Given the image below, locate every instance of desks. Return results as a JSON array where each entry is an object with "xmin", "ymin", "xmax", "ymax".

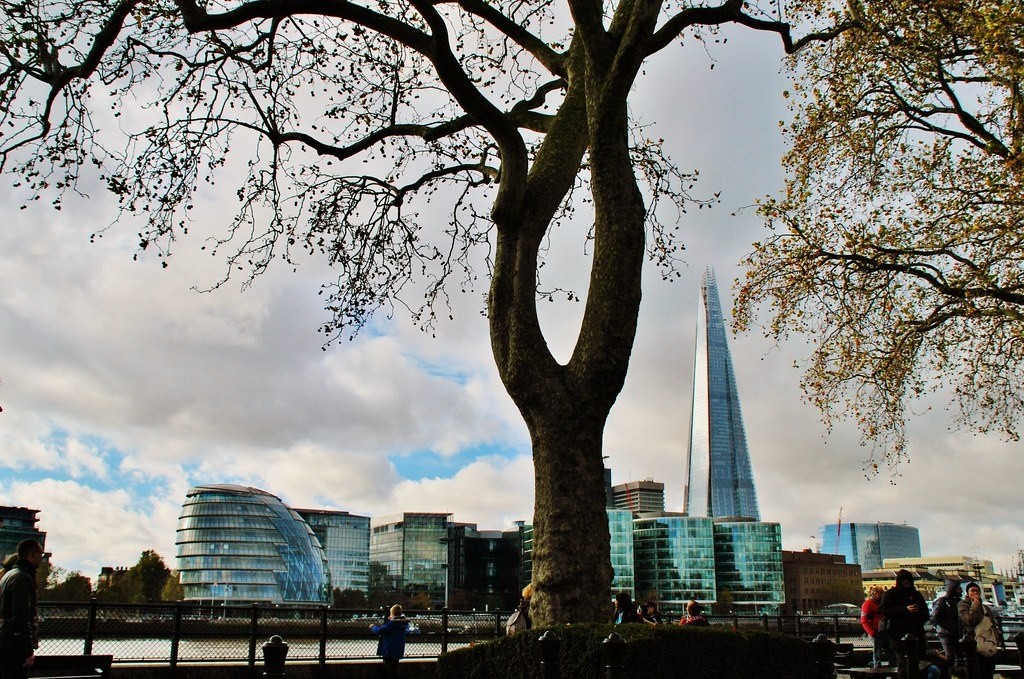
[{"xmin": 837, "ymin": 666, "xmax": 899, "ymax": 679}]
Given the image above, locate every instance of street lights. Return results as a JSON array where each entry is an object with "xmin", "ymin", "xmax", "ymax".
[{"xmin": 438, "ymin": 541, "xmax": 448, "ymax": 607}]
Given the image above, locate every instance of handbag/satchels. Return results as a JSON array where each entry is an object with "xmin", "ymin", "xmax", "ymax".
[{"xmin": 974, "ymin": 614, "xmax": 997, "ymax": 658}]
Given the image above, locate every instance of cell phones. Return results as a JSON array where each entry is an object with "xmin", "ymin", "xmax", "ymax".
[{"xmin": 913, "ymin": 604, "xmax": 920, "ymax": 608}]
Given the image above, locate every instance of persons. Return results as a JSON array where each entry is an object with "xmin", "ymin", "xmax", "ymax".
[
  {"xmin": 878, "ymin": 569, "xmax": 930, "ymax": 679},
  {"xmin": 505, "ymin": 583, "xmax": 533, "ymax": 636},
  {"xmin": 369, "ymin": 604, "xmax": 410, "ymax": 679},
  {"xmin": 679, "ymin": 599, "xmax": 705, "ymax": 627},
  {"xmin": 612, "ymin": 593, "xmax": 663, "ymax": 625},
  {"xmin": 0, "ymin": 538, "xmax": 45, "ymax": 679},
  {"xmin": 926, "ymin": 649, "xmax": 951, "ymax": 679},
  {"xmin": 861, "ymin": 587, "xmax": 896, "ymax": 667},
  {"xmin": 958, "ymin": 583, "xmax": 1000, "ymax": 679},
  {"xmin": 929, "ymin": 579, "xmax": 966, "ymax": 679}
]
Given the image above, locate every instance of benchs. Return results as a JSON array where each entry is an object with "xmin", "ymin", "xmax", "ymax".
[{"xmin": 27, "ymin": 655, "xmax": 113, "ymax": 679}]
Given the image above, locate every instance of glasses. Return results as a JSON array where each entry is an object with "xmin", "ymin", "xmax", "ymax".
[
  {"xmin": 957, "ymin": 587, "xmax": 962, "ymax": 590},
  {"xmin": 971, "ymin": 589, "xmax": 979, "ymax": 593},
  {"xmin": 899, "ymin": 576, "xmax": 915, "ymax": 582},
  {"xmin": 38, "ymin": 551, "xmax": 45, "ymax": 558}
]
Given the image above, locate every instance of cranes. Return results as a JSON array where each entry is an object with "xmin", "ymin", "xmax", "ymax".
[{"xmin": 834, "ymin": 507, "xmax": 843, "ymax": 553}]
